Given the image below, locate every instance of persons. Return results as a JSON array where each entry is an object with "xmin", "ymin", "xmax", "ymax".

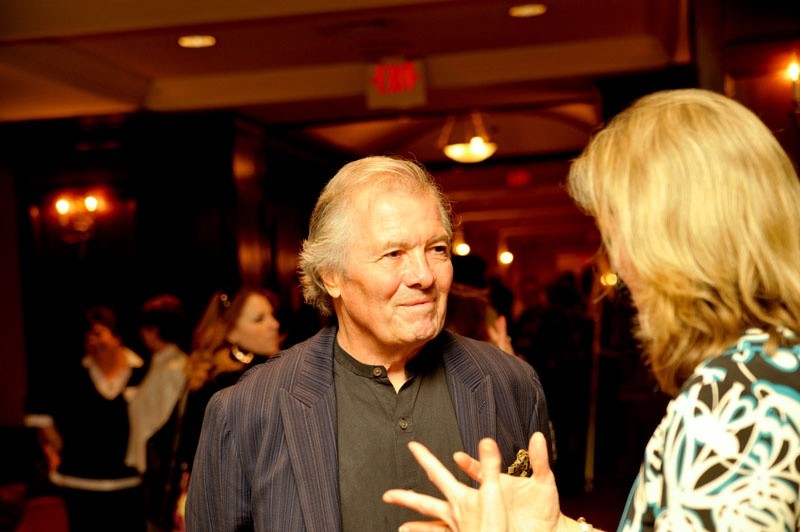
[
  {"xmin": 381, "ymin": 89, "xmax": 800, "ymax": 532},
  {"xmin": 185, "ymin": 156, "xmax": 550, "ymax": 532},
  {"xmin": 0, "ymin": 255, "xmax": 612, "ymax": 532}
]
